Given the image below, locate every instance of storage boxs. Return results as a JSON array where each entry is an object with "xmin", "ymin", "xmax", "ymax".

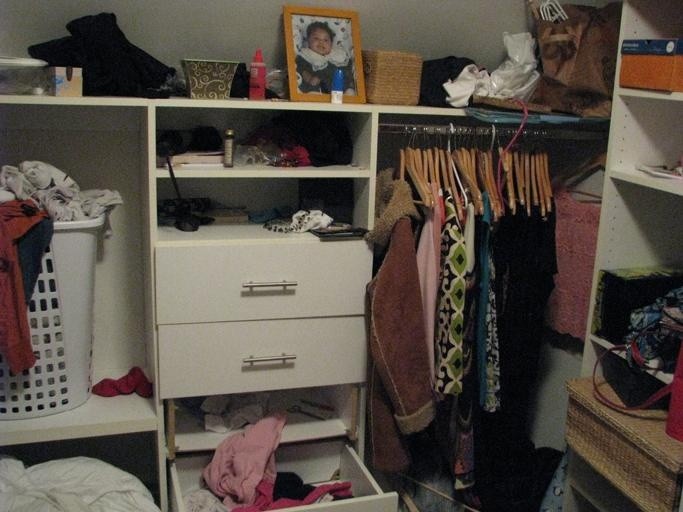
[
  {"xmin": 618, "ymin": 39, "xmax": 682, "ymax": 92},
  {"xmin": 565, "ymin": 376, "xmax": 681, "ymax": 511}
]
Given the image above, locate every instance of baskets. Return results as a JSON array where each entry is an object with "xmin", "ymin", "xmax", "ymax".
[
  {"xmin": 0, "ymin": 210, "xmax": 107, "ymax": 421},
  {"xmin": 361, "ymin": 49, "xmax": 423, "ymax": 106}
]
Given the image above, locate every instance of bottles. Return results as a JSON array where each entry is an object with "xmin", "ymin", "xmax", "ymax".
[
  {"xmin": 330, "ymin": 69, "xmax": 344, "ymax": 104},
  {"xmin": 225, "ymin": 129, "xmax": 234, "ymax": 167},
  {"xmin": 250, "ymin": 48, "xmax": 266, "ymax": 100}
]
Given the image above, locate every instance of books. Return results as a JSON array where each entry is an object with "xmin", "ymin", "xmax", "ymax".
[
  {"xmin": 172, "ymin": 148, "xmax": 226, "ymax": 169},
  {"xmin": 205, "ymin": 208, "xmax": 247, "ymax": 224}
]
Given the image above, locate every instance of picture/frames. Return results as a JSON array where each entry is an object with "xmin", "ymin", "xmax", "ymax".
[{"xmin": 283, "ymin": 5, "xmax": 367, "ymax": 104}]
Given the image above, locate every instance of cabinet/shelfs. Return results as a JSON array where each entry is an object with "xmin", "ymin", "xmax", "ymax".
[
  {"xmin": 1, "ymin": 95, "xmax": 398, "ymax": 509},
  {"xmin": 561, "ymin": 0, "xmax": 682, "ymax": 511}
]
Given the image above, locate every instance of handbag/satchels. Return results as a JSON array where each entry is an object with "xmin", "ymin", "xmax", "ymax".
[{"xmin": 536, "ymin": 0, "xmax": 624, "ymax": 119}]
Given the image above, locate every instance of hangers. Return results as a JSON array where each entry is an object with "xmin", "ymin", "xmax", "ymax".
[{"xmin": 400, "ymin": 125, "xmax": 553, "ymax": 221}]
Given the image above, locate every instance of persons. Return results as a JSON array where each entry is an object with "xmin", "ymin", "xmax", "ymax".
[{"xmin": 296, "ymin": 21, "xmax": 352, "ymax": 91}]
